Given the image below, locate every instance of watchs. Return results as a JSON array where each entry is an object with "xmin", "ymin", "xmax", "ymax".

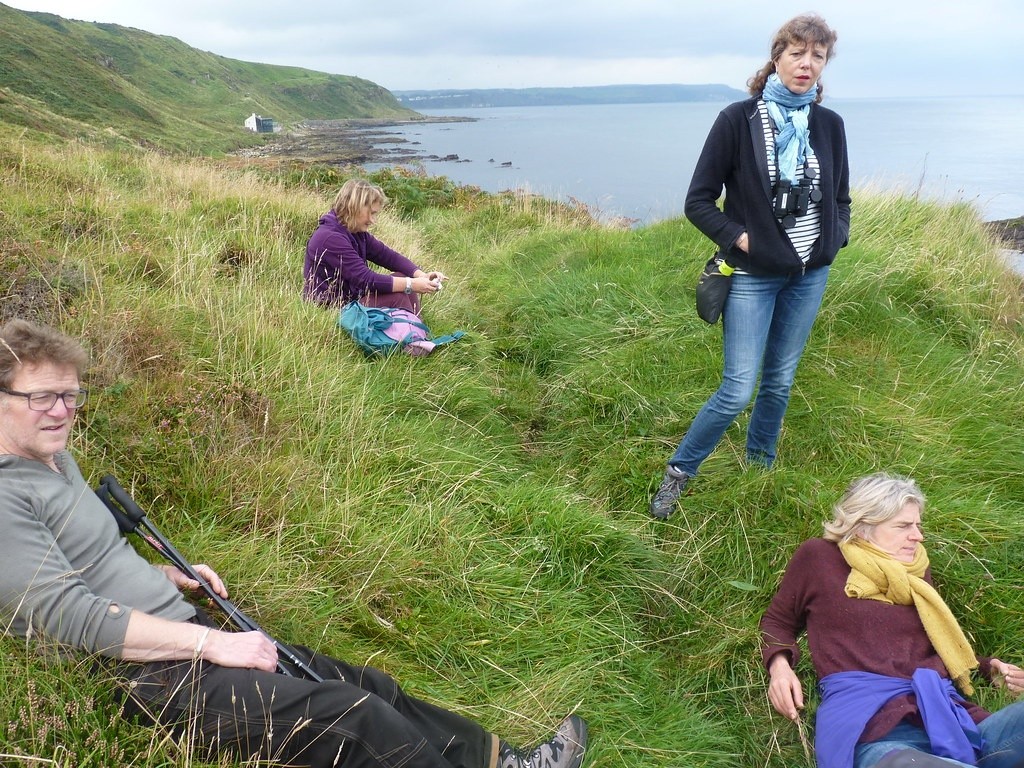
[{"xmin": 405, "ymin": 276, "xmax": 412, "ymax": 295}]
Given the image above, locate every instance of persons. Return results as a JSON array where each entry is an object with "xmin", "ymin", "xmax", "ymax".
[
  {"xmin": 306, "ymin": 177, "xmax": 451, "ymax": 320},
  {"xmin": 758, "ymin": 473, "xmax": 1024, "ymax": 768},
  {"xmin": 653, "ymin": 17, "xmax": 853, "ymax": 515},
  {"xmin": 0, "ymin": 319, "xmax": 586, "ymax": 768}
]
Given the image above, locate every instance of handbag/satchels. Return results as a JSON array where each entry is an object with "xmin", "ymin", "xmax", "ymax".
[{"xmin": 696, "ymin": 252, "xmax": 736, "ymax": 324}]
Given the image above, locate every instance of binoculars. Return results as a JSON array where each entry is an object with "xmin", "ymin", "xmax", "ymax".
[{"xmin": 773, "ymin": 178, "xmax": 811, "ymax": 219}]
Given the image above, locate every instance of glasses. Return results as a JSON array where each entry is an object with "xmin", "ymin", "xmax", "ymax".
[{"xmin": 0, "ymin": 387, "xmax": 86, "ymax": 412}]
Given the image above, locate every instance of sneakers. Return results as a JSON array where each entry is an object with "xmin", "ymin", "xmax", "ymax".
[
  {"xmin": 652, "ymin": 464, "xmax": 690, "ymax": 519},
  {"xmin": 497, "ymin": 712, "xmax": 587, "ymax": 768}
]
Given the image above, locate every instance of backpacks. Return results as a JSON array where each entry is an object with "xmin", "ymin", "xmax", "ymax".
[{"xmin": 340, "ymin": 299, "xmax": 432, "ymax": 359}]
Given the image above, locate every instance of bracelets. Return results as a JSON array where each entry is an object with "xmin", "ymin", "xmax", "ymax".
[{"xmin": 196, "ymin": 628, "xmax": 211, "ymax": 657}]
